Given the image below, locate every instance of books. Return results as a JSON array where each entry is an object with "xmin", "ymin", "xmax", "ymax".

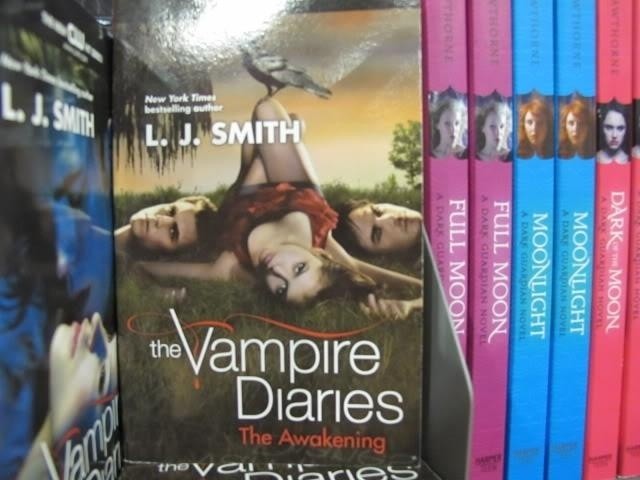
[
  {"xmin": 423, "ymin": 3, "xmax": 472, "ymax": 480},
  {"xmin": 110, "ymin": 2, "xmax": 423, "ymax": 480},
  {"xmin": 470, "ymin": 2, "xmax": 513, "ymax": 478},
  {"xmin": 584, "ymin": 0, "xmax": 639, "ymax": 479},
  {"xmin": 508, "ymin": 1, "xmax": 556, "ymax": 479},
  {"xmin": 545, "ymin": 2, "xmax": 598, "ymax": 479},
  {"xmin": 2, "ymin": 1, "xmax": 121, "ymax": 480}
]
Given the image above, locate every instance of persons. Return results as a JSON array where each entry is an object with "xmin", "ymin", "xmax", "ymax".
[
  {"xmin": 557, "ymin": 97, "xmax": 595, "ymax": 160},
  {"xmin": 3, "ymin": 143, "xmax": 113, "ymax": 477},
  {"xmin": 518, "ymin": 99, "xmax": 554, "ymax": 160},
  {"xmin": 429, "ymin": 99, "xmax": 467, "ymax": 159},
  {"xmin": 138, "ymin": 98, "xmax": 422, "ymax": 319},
  {"xmin": 16, "ymin": 312, "xmax": 118, "ymax": 479},
  {"xmin": 475, "ymin": 102, "xmax": 512, "ymax": 161},
  {"xmin": 114, "ymin": 195, "xmax": 217, "ymax": 262},
  {"xmin": 598, "ymin": 101, "xmax": 629, "ymax": 162},
  {"xmin": 332, "ymin": 200, "xmax": 422, "ymax": 277}
]
